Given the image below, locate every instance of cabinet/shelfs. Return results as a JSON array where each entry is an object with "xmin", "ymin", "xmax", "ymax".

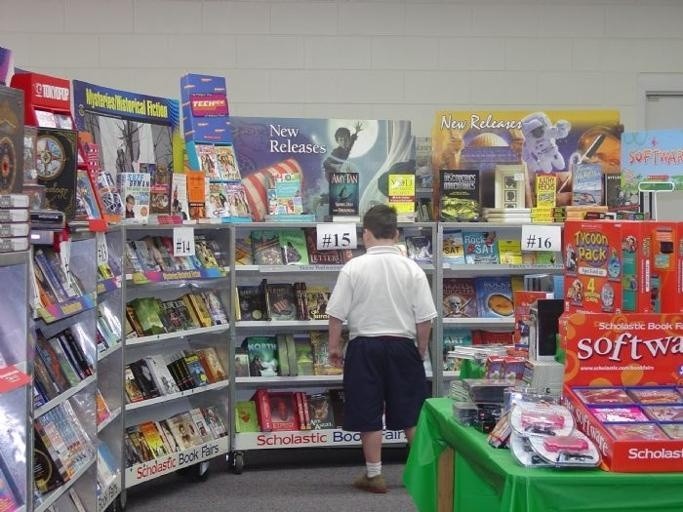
[
  {"xmin": 123, "ymin": 222, "xmax": 233, "ymax": 511},
  {"xmin": 231, "ymin": 223, "xmax": 438, "ymax": 473},
  {"xmin": 435, "ymin": 222, "xmax": 562, "ymax": 399},
  {"xmin": 97, "ymin": 222, "xmax": 124, "ymax": 511},
  {"xmin": 1, "ymin": 254, "xmax": 31, "ymax": 512},
  {"xmin": 30, "ymin": 231, "xmax": 97, "ymax": 512}
]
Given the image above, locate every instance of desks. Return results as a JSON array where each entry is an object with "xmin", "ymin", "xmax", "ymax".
[{"xmin": 423, "ymin": 397, "xmax": 683, "ymax": 511}]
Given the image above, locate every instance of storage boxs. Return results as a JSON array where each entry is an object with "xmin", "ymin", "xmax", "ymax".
[{"xmin": 447, "ymin": 343, "xmax": 529, "ymax": 433}]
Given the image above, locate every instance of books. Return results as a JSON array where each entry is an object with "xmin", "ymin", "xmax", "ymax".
[{"xmin": 0, "ymin": 85, "xmax": 95, "ymax": 488}]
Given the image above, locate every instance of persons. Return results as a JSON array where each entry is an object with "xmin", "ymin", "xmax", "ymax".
[
  {"xmin": 324, "ymin": 206, "xmax": 439, "ymax": 493},
  {"xmin": 322, "ymin": 121, "xmax": 364, "ymax": 187}
]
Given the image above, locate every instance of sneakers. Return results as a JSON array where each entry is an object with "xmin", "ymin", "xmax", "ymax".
[{"xmin": 354, "ymin": 474, "xmax": 386, "ymax": 492}]
